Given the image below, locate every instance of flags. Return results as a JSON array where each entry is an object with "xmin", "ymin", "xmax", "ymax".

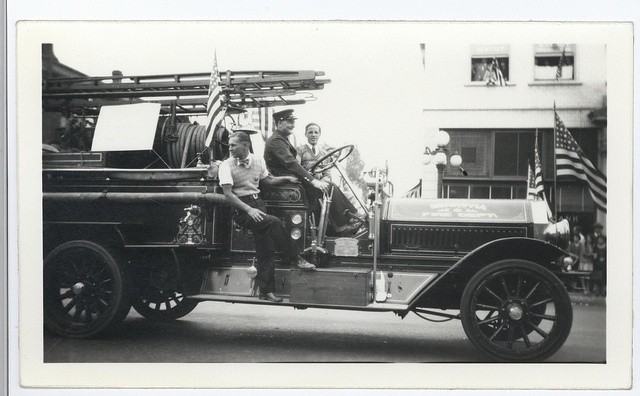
[
  {"xmin": 555, "ymin": 112, "xmax": 607, "ymax": 213},
  {"xmin": 528, "ymin": 163, "xmax": 538, "ymax": 200},
  {"xmin": 402, "ymin": 182, "xmax": 420, "ymax": 198},
  {"xmin": 204, "ymin": 48, "xmax": 228, "ymax": 149},
  {"xmin": 534, "ymin": 142, "xmax": 552, "ymax": 220},
  {"xmin": 484, "ymin": 60, "xmax": 507, "ymax": 87}
]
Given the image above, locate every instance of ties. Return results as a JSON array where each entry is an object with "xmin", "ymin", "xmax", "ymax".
[{"xmin": 311, "ymin": 146, "xmax": 315, "ymax": 154}]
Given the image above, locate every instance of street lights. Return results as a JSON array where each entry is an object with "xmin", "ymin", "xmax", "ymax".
[{"xmin": 421, "ymin": 130, "xmax": 463, "ymax": 198}]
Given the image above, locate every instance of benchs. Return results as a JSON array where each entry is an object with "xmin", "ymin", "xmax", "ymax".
[
  {"xmin": 568, "ymin": 271, "xmax": 591, "ymax": 295},
  {"xmin": 250, "ymin": 129, "xmax": 309, "ymax": 205}
]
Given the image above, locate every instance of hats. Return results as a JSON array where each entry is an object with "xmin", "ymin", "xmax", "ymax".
[
  {"xmin": 591, "ymin": 222, "xmax": 603, "ymax": 229},
  {"xmin": 272, "ymin": 108, "xmax": 300, "ymax": 120}
]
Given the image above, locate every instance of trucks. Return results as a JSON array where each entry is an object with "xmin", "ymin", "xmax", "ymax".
[{"xmin": 44, "ymin": 127, "xmax": 584, "ymax": 361}]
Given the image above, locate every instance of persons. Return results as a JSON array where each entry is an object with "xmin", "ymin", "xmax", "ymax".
[
  {"xmin": 218, "ymin": 131, "xmax": 316, "ymax": 302},
  {"xmin": 263, "ymin": 109, "xmax": 363, "ymax": 237},
  {"xmin": 295, "ymin": 123, "xmax": 358, "ymax": 228}
]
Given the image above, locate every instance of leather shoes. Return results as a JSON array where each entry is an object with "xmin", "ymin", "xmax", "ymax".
[
  {"xmin": 259, "ymin": 292, "xmax": 283, "ymax": 302},
  {"xmin": 290, "ymin": 254, "xmax": 316, "ymax": 270},
  {"xmin": 335, "ymin": 221, "xmax": 363, "ymax": 235}
]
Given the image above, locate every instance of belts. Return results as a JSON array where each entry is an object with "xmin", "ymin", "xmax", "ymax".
[{"xmin": 237, "ymin": 194, "xmax": 261, "ymax": 200}]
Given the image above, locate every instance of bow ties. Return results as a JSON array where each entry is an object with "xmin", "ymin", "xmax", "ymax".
[{"xmin": 237, "ymin": 157, "xmax": 251, "ymax": 168}]
